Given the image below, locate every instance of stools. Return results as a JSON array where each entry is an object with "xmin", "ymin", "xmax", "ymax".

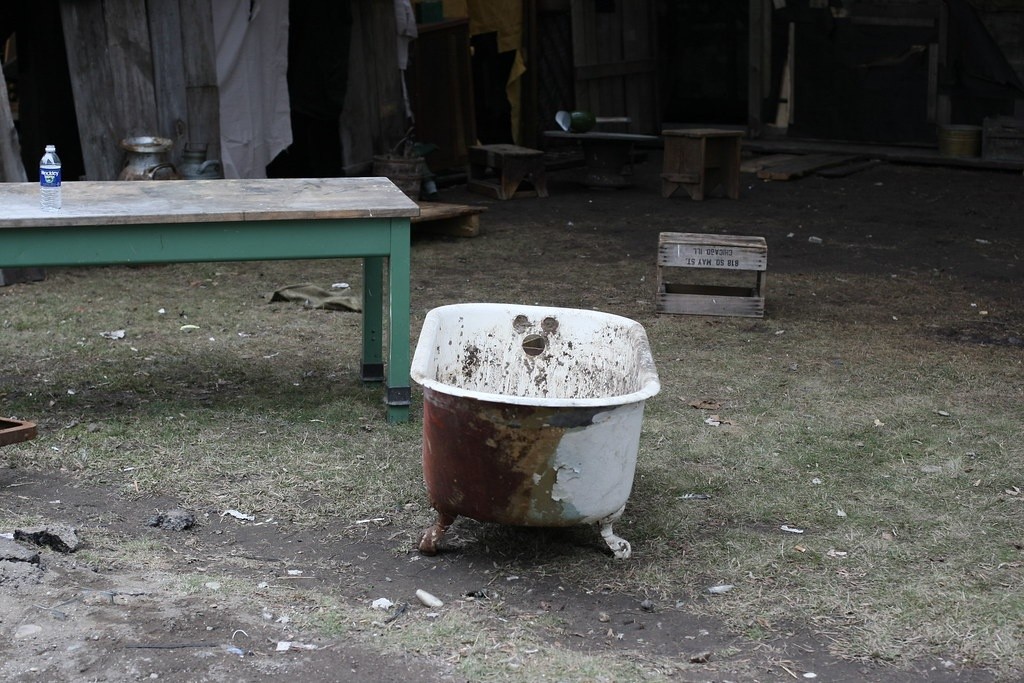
[
  {"xmin": 657, "ymin": 128, "xmax": 744, "ymax": 200},
  {"xmin": 464, "ymin": 144, "xmax": 544, "ymax": 198}
]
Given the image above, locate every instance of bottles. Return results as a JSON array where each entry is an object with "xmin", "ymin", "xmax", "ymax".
[
  {"xmin": 39, "ymin": 145, "xmax": 62, "ymax": 214},
  {"xmin": 117, "ymin": 135, "xmax": 181, "ymax": 180}
]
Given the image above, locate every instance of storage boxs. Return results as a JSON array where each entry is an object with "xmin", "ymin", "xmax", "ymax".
[
  {"xmin": 657, "ymin": 231, "xmax": 768, "ymax": 319},
  {"xmin": 981, "ymin": 118, "xmax": 1024, "ymax": 160}
]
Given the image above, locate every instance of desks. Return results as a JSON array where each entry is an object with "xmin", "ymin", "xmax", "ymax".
[
  {"xmin": 0, "ymin": 178, "xmax": 422, "ymax": 425},
  {"xmin": 544, "ymin": 131, "xmax": 658, "ymax": 189}
]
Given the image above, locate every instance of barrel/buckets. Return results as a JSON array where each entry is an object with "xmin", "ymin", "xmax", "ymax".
[{"xmin": 937, "ymin": 124, "xmax": 982, "ymax": 161}]
